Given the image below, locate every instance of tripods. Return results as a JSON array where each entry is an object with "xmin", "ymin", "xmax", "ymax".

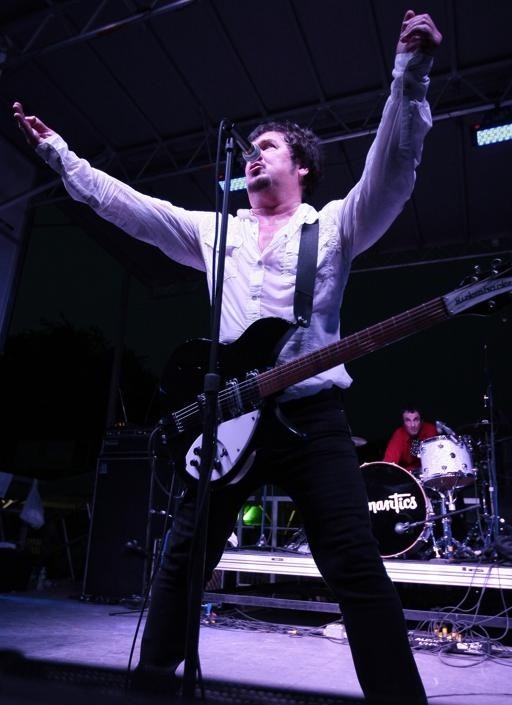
[
  {"xmin": 420, "ymin": 498, "xmax": 477, "ymax": 561},
  {"xmin": 451, "ymin": 466, "xmax": 512, "ymax": 561}
]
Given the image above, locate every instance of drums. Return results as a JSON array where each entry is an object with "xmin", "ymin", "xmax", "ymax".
[
  {"xmin": 417, "ymin": 435, "xmax": 475, "ymax": 491},
  {"xmin": 360, "ymin": 461, "xmax": 434, "ymax": 559}
]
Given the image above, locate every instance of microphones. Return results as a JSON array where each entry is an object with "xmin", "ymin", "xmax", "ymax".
[{"xmin": 224, "ymin": 123, "xmax": 260, "ymax": 160}]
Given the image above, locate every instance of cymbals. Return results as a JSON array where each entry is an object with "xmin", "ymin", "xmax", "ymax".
[{"xmin": 456, "ymin": 423, "xmax": 511, "ymax": 434}]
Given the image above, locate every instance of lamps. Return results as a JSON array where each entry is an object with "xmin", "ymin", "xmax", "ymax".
[
  {"xmin": 218, "ymin": 175, "xmax": 249, "ymax": 192},
  {"xmin": 473, "ymin": 105, "xmax": 512, "ymax": 149}
]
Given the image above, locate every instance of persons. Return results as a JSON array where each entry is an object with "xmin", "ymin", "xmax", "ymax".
[
  {"xmin": 13, "ymin": 8, "xmax": 442, "ymax": 705},
  {"xmin": 384, "ymin": 403, "xmax": 437, "ymax": 474}
]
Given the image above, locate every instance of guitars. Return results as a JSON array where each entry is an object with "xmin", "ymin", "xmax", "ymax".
[{"xmin": 165, "ymin": 259, "xmax": 511, "ymax": 485}]
